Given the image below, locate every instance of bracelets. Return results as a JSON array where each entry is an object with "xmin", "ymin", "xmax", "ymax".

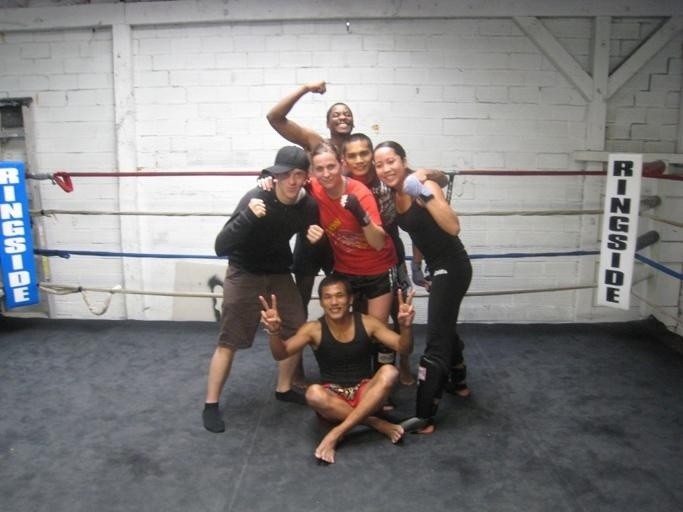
[{"xmin": 264, "ymin": 328, "xmax": 281, "ymax": 336}]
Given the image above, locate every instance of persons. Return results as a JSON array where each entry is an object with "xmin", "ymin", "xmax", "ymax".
[
  {"xmin": 258, "ymin": 142, "xmax": 399, "ymax": 411},
  {"xmin": 201, "ymin": 147, "xmax": 335, "ymax": 433},
  {"xmin": 266, "ymin": 80, "xmax": 356, "ymax": 391},
  {"xmin": 340, "ymin": 132, "xmax": 450, "ymax": 386},
  {"xmin": 256, "ymin": 273, "xmax": 418, "ymax": 464},
  {"xmin": 371, "ymin": 142, "xmax": 474, "ymax": 434}
]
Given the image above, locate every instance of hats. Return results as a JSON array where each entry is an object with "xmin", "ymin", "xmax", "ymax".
[{"xmin": 262, "ymin": 146, "xmax": 308, "ymax": 176}]
